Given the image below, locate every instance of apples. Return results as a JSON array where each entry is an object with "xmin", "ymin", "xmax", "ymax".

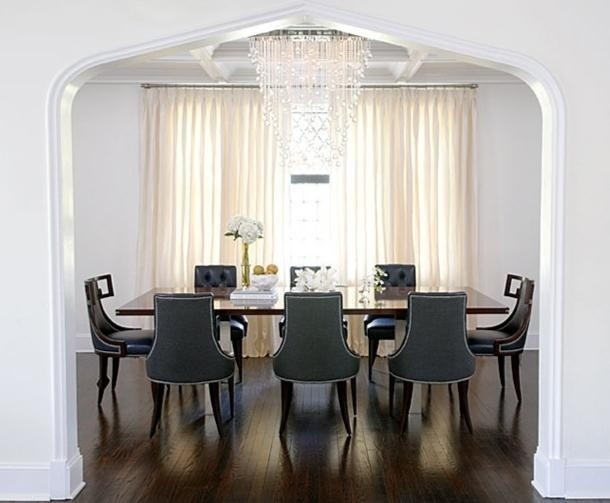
[{"xmin": 254, "ymin": 264, "xmax": 278, "ymax": 274}]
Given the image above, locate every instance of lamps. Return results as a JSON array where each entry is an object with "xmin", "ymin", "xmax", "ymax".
[{"xmin": 245, "ymin": 31, "xmax": 374, "ymax": 174}]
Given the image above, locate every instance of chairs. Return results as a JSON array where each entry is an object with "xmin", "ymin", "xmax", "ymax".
[
  {"xmin": 385, "ymin": 291, "xmax": 473, "ymax": 437},
  {"xmin": 145, "ymin": 292, "xmax": 237, "ymax": 437},
  {"xmin": 194, "ymin": 265, "xmax": 249, "ymax": 383},
  {"xmin": 268, "ymin": 291, "xmax": 361, "ymax": 437},
  {"xmin": 81, "ymin": 271, "xmax": 155, "ymax": 406},
  {"xmin": 278, "ymin": 266, "xmax": 349, "ymax": 339},
  {"xmin": 448, "ymin": 274, "xmax": 535, "ymax": 406},
  {"xmin": 363, "ymin": 264, "xmax": 418, "ymax": 383}
]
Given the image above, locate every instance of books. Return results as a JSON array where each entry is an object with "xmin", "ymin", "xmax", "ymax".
[
  {"xmin": 195, "ymin": 286, "xmax": 237, "ymax": 300},
  {"xmin": 229, "ymin": 286, "xmax": 279, "ymax": 300}
]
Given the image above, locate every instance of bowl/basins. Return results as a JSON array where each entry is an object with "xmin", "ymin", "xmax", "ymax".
[{"xmin": 251, "ymin": 273, "xmax": 278, "ymax": 292}]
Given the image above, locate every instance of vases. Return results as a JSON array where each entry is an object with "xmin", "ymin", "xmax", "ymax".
[{"xmin": 240, "ymin": 265, "xmax": 252, "ymax": 285}]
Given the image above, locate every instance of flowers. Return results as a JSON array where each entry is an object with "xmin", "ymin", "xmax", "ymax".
[{"xmin": 225, "ymin": 212, "xmax": 267, "ymax": 287}]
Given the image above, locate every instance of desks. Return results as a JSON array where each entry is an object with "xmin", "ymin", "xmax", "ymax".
[{"xmin": 113, "ymin": 287, "xmax": 511, "ymax": 415}]
